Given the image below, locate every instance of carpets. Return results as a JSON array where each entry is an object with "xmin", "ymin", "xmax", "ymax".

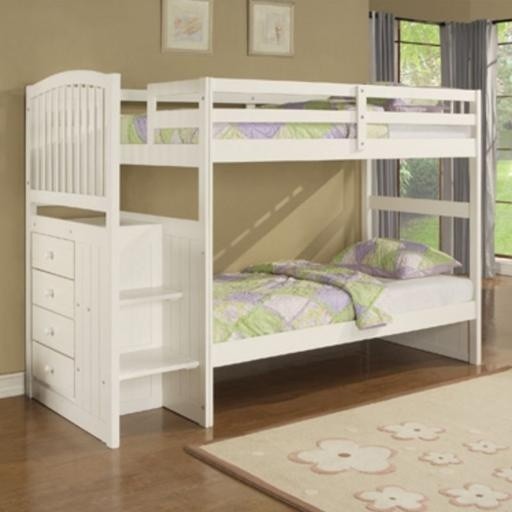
[{"xmin": 178, "ymin": 365, "xmax": 512, "ymax": 512}]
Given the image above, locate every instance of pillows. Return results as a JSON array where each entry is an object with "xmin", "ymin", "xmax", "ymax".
[
  {"xmin": 326, "ymin": 79, "xmax": 455, "ymax": 115},
  {"xmin": 325, "ymin": 236, "xmax": 465, "ymax": 282}
]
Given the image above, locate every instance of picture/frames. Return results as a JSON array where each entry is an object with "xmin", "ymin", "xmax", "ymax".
[
  {"xmin": 246, "ymin": 1, "xmax": 294, "ymax": 57},
  {"xmin": 159, "ymin": 0, "xmax": 214, "ymax": 54}
]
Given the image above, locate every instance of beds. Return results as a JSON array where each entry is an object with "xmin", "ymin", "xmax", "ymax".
[{"xmin": 21, "ymin": 67, "xmax": 485, "ymax": 451}]
